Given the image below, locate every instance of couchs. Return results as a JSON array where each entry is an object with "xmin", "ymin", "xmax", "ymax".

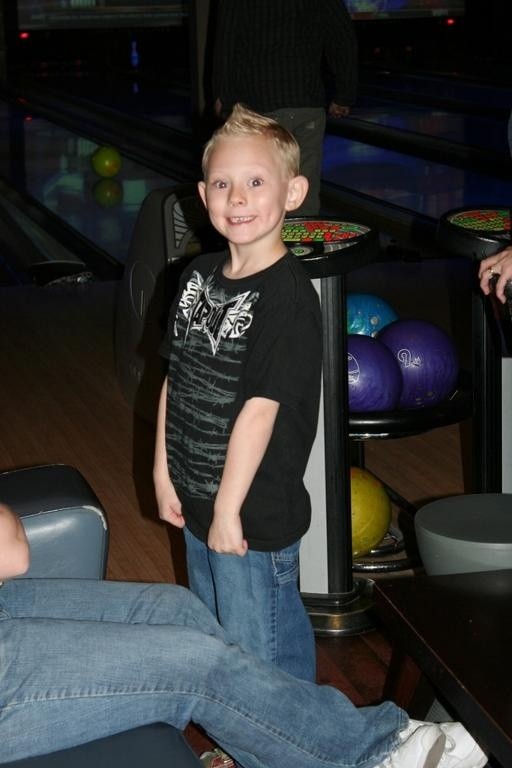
[{"xmin": 0, "ymin": 459, "xmax": 205, "ymax": 768}]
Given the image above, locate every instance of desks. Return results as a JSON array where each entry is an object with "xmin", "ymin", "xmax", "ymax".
[{"xmin": 369, "ymin": 568, "xmax": 512, "ymax": 768}]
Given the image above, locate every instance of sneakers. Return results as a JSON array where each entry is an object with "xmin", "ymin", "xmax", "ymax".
[
  {"xmin": 381, "ymin": 725, "xmax": 444, "ymax": 768},
  {"xmin": 406, "ymin": 710, "xmax": 489, "ymax": 768}
]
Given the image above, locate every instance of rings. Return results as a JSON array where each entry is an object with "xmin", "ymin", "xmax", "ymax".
[{"xmin": 486, "ymin": 264, "xmax": 496, "ymax": 280}]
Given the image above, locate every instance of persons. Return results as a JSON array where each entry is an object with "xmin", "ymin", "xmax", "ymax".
[
  {"xmin": 152, "ymin": 102, "xmax": 325, "ymax": 682},
  {"xmin": 478, "ymin": 244, "xmax": 511, "ymax": 304},
  {"xmin": 0, "ymin": 498, "xmax": 492, "ymax": 768}
]
